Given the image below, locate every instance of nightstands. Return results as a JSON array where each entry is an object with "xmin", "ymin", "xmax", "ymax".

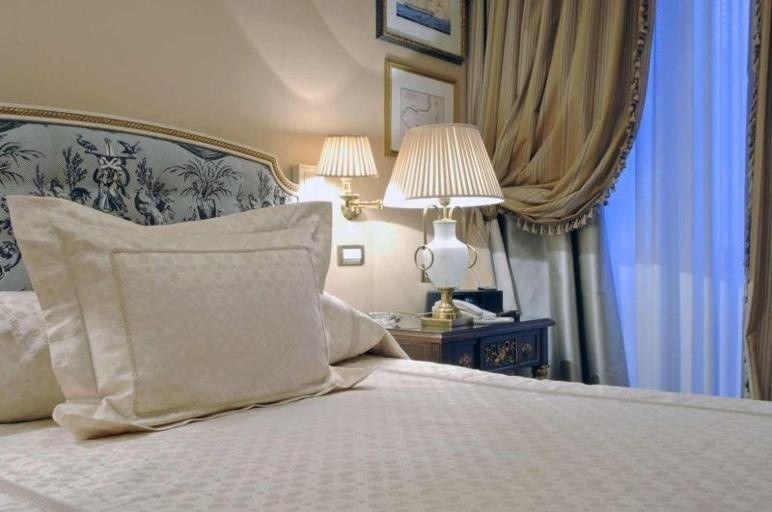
[{"xmin": 373, "ymin": 312, "xmax": 556, "ymax": 381}]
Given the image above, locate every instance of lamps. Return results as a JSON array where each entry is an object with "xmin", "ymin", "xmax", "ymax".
[
  {"xmin": 315, "ymin": 134, "xmax": 383, "ymax": 222},
  {"xmin": 381, "ymin": 122, "xmax": 504, "ymax": 329}
]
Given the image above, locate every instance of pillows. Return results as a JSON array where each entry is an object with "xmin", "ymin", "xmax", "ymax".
[
  {"xmin": 4, "ymin": 193, "xmax": 382, "ymax": 441},
  {"xmin": 0, "ymin": 290, "xmax": 414, "ymax": 425}
]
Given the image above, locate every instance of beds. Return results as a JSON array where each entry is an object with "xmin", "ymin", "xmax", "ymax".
[{"xmin": 0, "ymin": 103, "xmax": 770, "ymax": 512}]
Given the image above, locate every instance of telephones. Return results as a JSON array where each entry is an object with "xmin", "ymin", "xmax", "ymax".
[{"xmin": 432, "ymin": 298, "xmax": 496, "ymax": 320}]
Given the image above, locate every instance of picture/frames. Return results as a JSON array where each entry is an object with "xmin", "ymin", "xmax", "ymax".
[{"xmin": 374, "ymin": 0, "xmax": 468, "ymax": 165}]
